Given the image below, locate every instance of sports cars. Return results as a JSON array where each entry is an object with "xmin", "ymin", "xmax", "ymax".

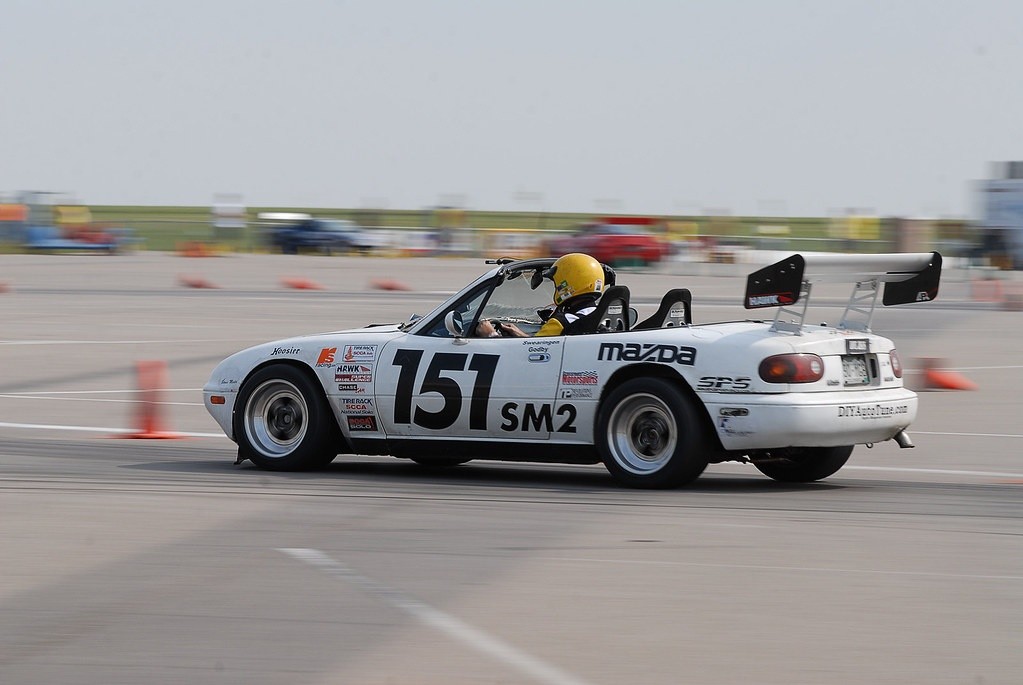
[{"xmin": 200, "ymin": 256, "xmax": 918, "ymax": 485}]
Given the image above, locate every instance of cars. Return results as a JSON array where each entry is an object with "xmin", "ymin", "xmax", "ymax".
[
  {"xmin": 264, "ymin": 216, "xmax": 371, "ymax": 254},
  {"xmin": 545, "ymin": 222, "xmax": 668, "ymax": 269}
]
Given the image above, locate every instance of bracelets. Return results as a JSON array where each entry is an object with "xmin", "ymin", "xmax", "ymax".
[{"xmin": 489, "ymin": 330, "xmax": 502, "ymax": 337}]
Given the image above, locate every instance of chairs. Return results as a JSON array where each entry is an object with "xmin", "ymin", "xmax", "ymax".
[
  {"xmin": 631, "ymin": 288, "xmax": 692, "ymax": 330},
  {"xmin": 560, "ymin": 286, "xmax": 630, "ymax": 337}
]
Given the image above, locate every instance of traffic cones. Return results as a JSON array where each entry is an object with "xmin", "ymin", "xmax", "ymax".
[
  {"xmin": 289, "ymin": 278, "xmax": 323, "ymax": 294},
  {"xmin": 911, "ymin": 353, "xmax": 976, "ymax": 390},
  {"xmin": 373, "ymin": 276, "xmax": 407, "ymax": 293},
  {"xmin": 184, "ymin": 275, "xmax": 218, "ymax": 292},
  {"xmin": 123, "ymin": 352, "xmax": 183, "ymax": 443}
]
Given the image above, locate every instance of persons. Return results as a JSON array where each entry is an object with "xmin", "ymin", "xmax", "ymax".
[{"xmin": 477, "ymin": 253, "xmax": 606, "ymax": 337}]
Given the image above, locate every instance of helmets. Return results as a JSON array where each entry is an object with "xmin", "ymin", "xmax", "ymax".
[{"xmin": 542, "ymin": 253, "xmax": 605, "ymax": 306}]
